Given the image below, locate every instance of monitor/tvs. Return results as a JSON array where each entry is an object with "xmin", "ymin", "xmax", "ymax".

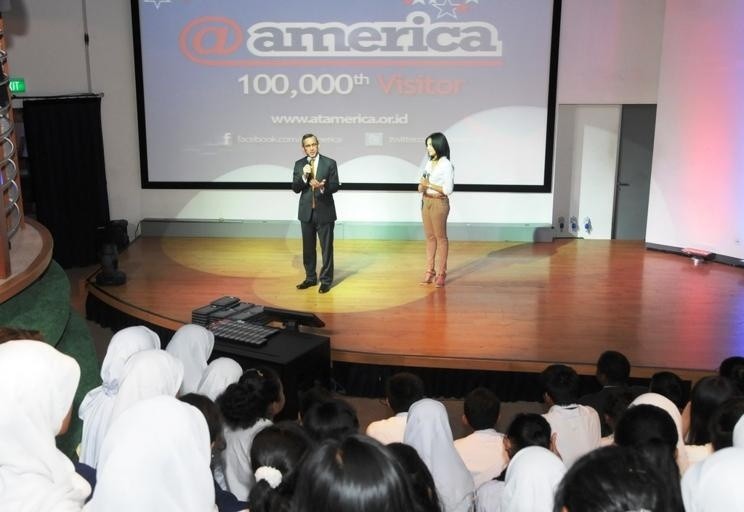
[{"xmin": 265, "ymin": 306, "xmax": 324, "ymax": 331}]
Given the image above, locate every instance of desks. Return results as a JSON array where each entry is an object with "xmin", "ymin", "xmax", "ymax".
[{"xmin": 204, "ymin": 318, "xmax": 331, "ymax": 422}]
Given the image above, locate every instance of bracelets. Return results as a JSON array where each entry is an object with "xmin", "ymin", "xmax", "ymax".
[{"xmin": 428, "ymin": 183, "xmax": 434, "ymax": 190}]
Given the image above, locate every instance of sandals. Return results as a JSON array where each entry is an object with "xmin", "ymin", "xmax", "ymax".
[{"xmin": 420, "ymin": 270, "xmax": 446, "ymax": 288}]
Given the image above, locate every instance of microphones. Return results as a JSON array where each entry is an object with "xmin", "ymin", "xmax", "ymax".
[{"xmin": 305, "ymin": 156, "xmax": 312, "ymax": 180}]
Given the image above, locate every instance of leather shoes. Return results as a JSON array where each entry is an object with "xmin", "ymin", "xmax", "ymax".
[
  {"xmin": 297, "ymin": 281, "xmax": 317, "ymax": 289},
  {"xmin": 319, "ymin": 285, "xmax": 329, "ymax": 293}
]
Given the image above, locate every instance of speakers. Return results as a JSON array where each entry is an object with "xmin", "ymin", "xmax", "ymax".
[{"xmin": 22, "ymin": 97, "xmax": 110, "ymax": 227}]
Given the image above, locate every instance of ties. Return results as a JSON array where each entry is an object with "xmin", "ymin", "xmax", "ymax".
[{"xmin": 310, "ymin": 159, "xmax": 316, "ymax": 209}]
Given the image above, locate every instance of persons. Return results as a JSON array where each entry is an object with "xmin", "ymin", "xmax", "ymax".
[
  {"xmin": 291, "ymin": 134, "xmax": 339, "ymax": 296},
  {"xmin": 1, "ymin": 321, "xmax": 744, "ymax": 511},
  {"xmin": 416, "ymin": 131, "xmax": 456, "ymax": 287}
]
